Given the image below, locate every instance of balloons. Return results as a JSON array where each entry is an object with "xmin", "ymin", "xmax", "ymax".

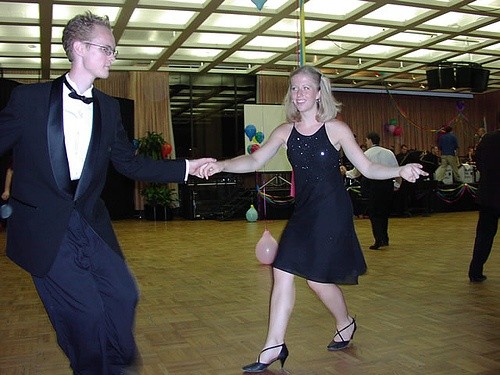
[
  {"xmin": 246, "ymin": 205, "xmax": 258, "ymax": 221},
  {"xmin": 251, "ymin": 145, "xmax": 260, "ymax": 153},
  {"xmin": 390, "ymin": 118, "xmax": 397, "ymax": 125},
  {"xmin": 256, "ymin": 132, "xmax": 264, "ymax": 144},
  {"xmin": 162, "ymin": 144, "xmax": 172, "ymax": 158},
  {"xmin": 388, "ymin": 125, "xmax": 396, "ymax": 132},
  {"xmin": 192, "ymin": 65, "xmax": 430, "ymax": 372},
  {"xmin": 252, "ymin": 136, "xmax": 259, "ymax": 144},
  {"xmin": 247, "ymin": 145, "xmax": 253, "ymax": 154},
  {"xmin": 251, "ymin": 0, "xmax": 267, "ymax": 10},
  {"xmin": 245, "ymin": 125, "xmax": 256, "ymax": 141},
  {"xmin": 393, "ymin": 127, "xmax": 401, "ymax": 136},
  {"xmin": 255, "ymin": 230, "xmax": 278, "ymax": 265}
]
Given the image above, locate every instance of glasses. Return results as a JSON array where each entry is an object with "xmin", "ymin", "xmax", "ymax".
[{"xmin": 84, "ymin": 42, "xmax": 118, "ymax": 57}]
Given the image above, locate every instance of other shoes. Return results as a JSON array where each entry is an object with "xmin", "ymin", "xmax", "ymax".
[
  {"xmin": 469, "ymin": 276, "xmax": 487, "ymax": 282},
  {"xmin": 369, "ymin": 239, "xmax": 389, "ymax": 250}
]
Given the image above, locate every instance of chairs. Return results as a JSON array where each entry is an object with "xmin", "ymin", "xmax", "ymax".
[{"xmin": 403, "ymin": 176, "xmax": 430, "ymax": 219}]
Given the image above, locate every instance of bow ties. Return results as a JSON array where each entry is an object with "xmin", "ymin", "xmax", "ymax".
[{"xmin": 62, "ymin": 75, "xmax": 95, "ymax": 105}]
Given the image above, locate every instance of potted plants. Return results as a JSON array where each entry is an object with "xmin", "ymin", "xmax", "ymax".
[{"xmin": 134, "ymin": 132, "xmax": 182, "ymax": 220}]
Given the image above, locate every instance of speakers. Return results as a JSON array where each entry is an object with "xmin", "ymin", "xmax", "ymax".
[
  {"xmin": 427, "ymin": 68, "xmax": 455, "ymax": 90},
  {"xmin": 457, "ymin": 67, "xmax": 490, "ymax": 92}
]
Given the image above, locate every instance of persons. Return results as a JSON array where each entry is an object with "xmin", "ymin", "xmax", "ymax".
[
  {"xmin": 340, "ymin": 133, "xmax": 400, "ymax": 250},
  {"xmin": 467, "ymin": 146, "xmax": 477, "ymax": 162},
  {"xmin": 0, "ymin": 11, "xmax": 218, "ymax": 375},
  {"xmin": 396, "ymin": 144, "xmax": 407, "ymax": 164},
  {"xmin": 425, "ymin": 145, "xmax": 439, "ymax": 166},
  {"xmin": 476, "ymin": 128, "xmax": 486, "ymax": 147},
  {"xmin": 468, "ymin": 112, "xmax": 500, "ymax": 281},
  {"xmin": 360, "ymin": 145, "xmax": 367, "ymax": 153},
  {"xmin": 437, "ymin": 127, "xmax": 465, "ymax": 183}
]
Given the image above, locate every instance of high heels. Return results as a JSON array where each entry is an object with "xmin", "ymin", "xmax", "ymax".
[
  {"xmin": 326, "ymin": 315, "xmax": 358, "ymax": 351},
  {"xmin": 241, "ymin": 342, "xmax": 289, "ymax": 373}
]
